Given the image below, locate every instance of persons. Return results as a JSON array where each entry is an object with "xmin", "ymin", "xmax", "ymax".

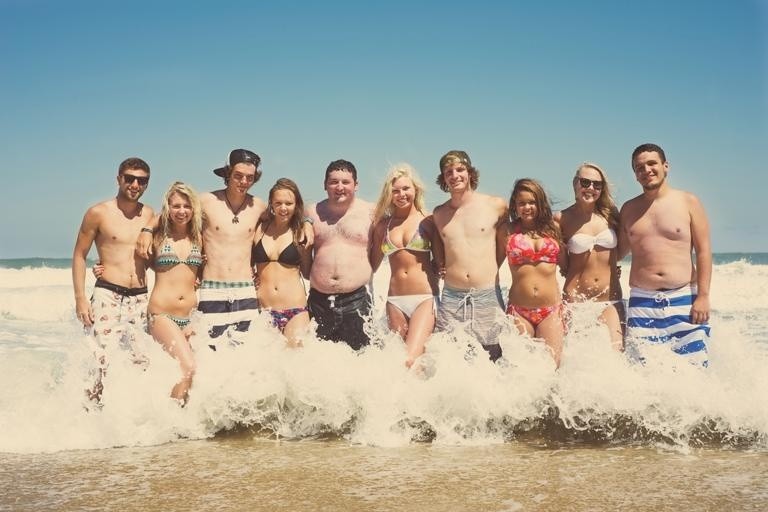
[
  {"xmin": 72, "ymin": 157, "xmax": 201, "ymax": 417},
  {"xmin": 497, "ymin": 177, "xmax": 621, "ymax": 368},
  {"xmin": 307, "ymin": 159, "xmax": 378, "ymax": 353},
  {"xmin": 94, "ymin": 181, "xmax": 206, "ymax": 411},
  {"xmin": 136, "ymin": 148, "xmax": 315, "ymax": 357},
  {"xmin": 562, "ymin": 142, "xmax": 713, "ymax": 374},
  {"xmin": 369, "ymin": 162, "xmax": 446, "ymax": 373},
  {"xmin": 551, "ymin": 162, "xmax": 627, "ymax": 352},
  {"xmin": 433, "ymin": 149, "xmax": 510, "ymax": 364},
  {"xmin": 200, "ymin": 178, "xmax": 313, "ymax": 354}
]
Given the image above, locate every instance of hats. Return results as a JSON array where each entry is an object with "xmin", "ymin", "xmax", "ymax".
[{"xmin": 212, "ymin": 147, "xmax": 262, "ymax": 183}]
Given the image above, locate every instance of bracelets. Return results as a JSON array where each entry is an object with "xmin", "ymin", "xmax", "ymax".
[{"xmin": 140, "ymin": 227, "xmax": 152, "ymax": 233}]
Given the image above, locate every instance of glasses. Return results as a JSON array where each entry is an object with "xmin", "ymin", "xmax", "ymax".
[
  {"xmin": 123, "ymin": 173, "xmax": 149, "ymax": 185},
  {"xmin": 579, "ymin": 176, "xmax": 603, "ymax": 191}
]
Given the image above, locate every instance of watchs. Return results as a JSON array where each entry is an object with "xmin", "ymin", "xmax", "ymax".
[{"xmin": 302, "ymin": 216, "xmax": 316, "ymax": 225}]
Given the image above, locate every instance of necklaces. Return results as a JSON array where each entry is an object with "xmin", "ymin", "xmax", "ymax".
[{"xmin": 221, "ymin": 188, "xmax": 248, "ymax": 225}]
